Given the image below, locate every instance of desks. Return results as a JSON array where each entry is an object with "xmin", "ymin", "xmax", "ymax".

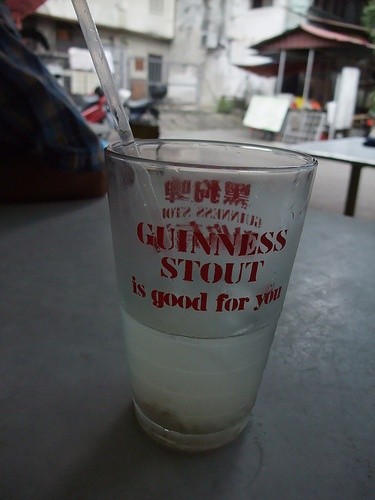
[{"xmin": 291, "ymin": 135, "xmax": 375, "ymax": 218}]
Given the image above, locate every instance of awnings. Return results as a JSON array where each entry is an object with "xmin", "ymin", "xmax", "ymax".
[{"xmin": 233, "ymin": 59, "xmax": 302, "ymax": 78}]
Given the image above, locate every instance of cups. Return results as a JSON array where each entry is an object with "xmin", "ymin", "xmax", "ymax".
[{"xmin": 104, "ymin": 139, "xmax": 318, "ymax": 452}]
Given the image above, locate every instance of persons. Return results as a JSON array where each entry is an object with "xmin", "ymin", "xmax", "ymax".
[{"xmin": 0, "ymin": 0, "xmax": 109, "ymax": 202}]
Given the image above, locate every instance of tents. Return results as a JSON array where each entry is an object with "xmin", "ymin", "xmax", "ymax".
[{"xmin": 249, "ymin": 24, "xmax": 374, "ymax": 103}]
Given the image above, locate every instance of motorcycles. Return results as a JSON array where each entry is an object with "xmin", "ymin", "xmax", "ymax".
[{"xmin": 72, "ymin": 80, "xmax": 170, "ymax": 127}]
